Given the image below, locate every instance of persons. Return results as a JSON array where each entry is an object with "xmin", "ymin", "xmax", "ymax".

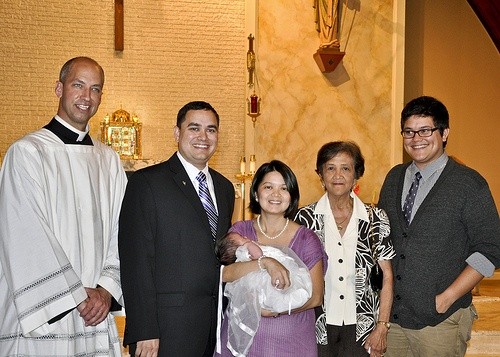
[
  {"xmin": 118, "ymin": 100, "xmax": 235, "ymax": 357},
  {"xmin": 293, "ymin": 140, "xmax": 396, "ymax": 357},
  {"xmin": 376, "ymin": 95, "xmax": 500, "ymax": 357},
  {"xmin": 221, "ymin": 159, "xmax": 324, "ymax": 357},
  {"xmin": 0, "ymin": 55, "xmax": 128, "ymax": 357},
  {"xmin": 214, "ymin": 232, "xmax": 310, "ymax": 311}
]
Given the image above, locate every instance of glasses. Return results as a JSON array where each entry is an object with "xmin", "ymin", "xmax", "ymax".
[{"xmin": 401, "ymin": 127, "xmax": 440, "ymax": 138}]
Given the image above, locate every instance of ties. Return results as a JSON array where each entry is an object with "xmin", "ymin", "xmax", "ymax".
[
  {"xmin": 194, "ymin": 170, "xmax": 217, "ymax": 242},
  {"xmin": 403, "ymin": 171, "xmax": 422, "ymax": 224}
]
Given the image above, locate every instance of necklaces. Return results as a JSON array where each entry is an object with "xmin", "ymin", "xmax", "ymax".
[
  {"xmin": 257, "ymin": 215, "xmax": 289, "ymax": 239},
  {"xmin": 335, "ymin": 204, "xmax": 353, "ymax": 229}
]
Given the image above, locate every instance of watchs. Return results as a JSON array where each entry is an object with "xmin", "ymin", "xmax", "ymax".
[{"xmin": 376, "ymin": 321, "xmax": 391, "ymax": 329}]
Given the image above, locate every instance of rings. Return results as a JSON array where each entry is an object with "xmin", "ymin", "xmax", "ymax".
[{"xmin": 275, "ymin": 280, "xmax": 279, "ymax": 286}]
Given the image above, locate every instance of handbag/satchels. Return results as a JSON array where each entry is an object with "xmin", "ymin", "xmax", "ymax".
[{"xmin": 368, "ymin": 206, "xmax": 384, "ymax": 292}]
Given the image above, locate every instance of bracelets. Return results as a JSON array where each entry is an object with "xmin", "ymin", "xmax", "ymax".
[
  {"xmin": 272, "ymin": 312, "xmax": 281, "ymax": 318},
  {"xmin": 258, "ymin": 255, "xmax": 266, "ymax": 271}
]
